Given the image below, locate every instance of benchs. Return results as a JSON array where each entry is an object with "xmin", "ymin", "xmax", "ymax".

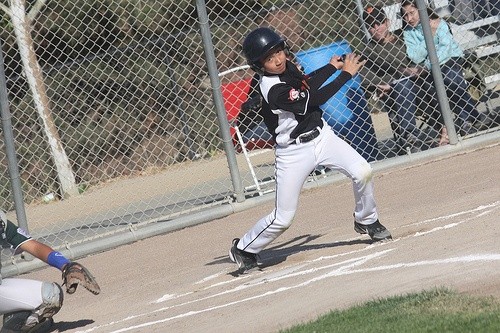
[{"xmin": 459, "ymin": 14, "xmax": 500, "ymax": 112}]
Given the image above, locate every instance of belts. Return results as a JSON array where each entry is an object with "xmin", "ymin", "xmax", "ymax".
[{"xmin": 289, "ymin": 121, "xmax": 324, "ymax": 145}]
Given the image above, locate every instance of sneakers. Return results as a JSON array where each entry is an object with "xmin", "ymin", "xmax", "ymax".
[
  {"xmin": 228, "ymin": 237, "xmax": 263, "ymax": 274},
  {"xmin": 352, "ymin": 213, "xmax": 392, "ymax": 244}
]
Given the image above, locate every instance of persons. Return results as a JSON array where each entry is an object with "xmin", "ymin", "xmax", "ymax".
[
  {"xmin": 0, "ymin": 216, "xmax": 100, "ymax": 333},
  {"xmin": 228, "ymin": 26, "xmax": 393, "ymax": 274},
  {"xmin": 350, "ymin": 0, "xmax": 482, "ymax": 154}
]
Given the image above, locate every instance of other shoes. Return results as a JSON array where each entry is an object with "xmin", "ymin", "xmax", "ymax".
[
  {"xmin": 439, "ymin": 127, "xmax": 451, "ymax": 146},
  {"xmin": 401, "ymin": 130, "xmax": 422, "ymax": 154}
]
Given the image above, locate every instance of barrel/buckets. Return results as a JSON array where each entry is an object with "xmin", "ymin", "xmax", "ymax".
[{"xmin": 293, "ymin": 40, "xmax": 380, "ymax": 162}]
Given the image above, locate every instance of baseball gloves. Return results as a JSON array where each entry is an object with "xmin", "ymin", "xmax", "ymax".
[{"xmin": 61, "ymin": 261, "xmax": 101, "ymax": 296}]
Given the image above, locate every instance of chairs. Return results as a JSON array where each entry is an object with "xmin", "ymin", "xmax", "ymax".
[{"xmin": 217, "ymin": 65, "xmax": 327, "ymax": 197}]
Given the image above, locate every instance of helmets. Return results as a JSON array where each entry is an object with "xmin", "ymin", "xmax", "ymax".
[{"xmin": 242, "ymin": 27, "xmax": 285, "ymax": 65}]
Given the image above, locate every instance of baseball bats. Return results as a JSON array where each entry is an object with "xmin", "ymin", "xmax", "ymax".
[{"xmin": 306, "ymin": 54, "xmax": 346, "ymax": 78}]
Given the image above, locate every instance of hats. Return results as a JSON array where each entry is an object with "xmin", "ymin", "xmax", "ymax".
[{"xmin": 362, "ymin": 5, "xmax": 386, "ymax": 22}]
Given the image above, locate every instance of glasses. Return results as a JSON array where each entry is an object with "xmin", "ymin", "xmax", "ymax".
[{"xmin": 365, "ymin": 18, "xmax": 386, "ymax": 30}]
